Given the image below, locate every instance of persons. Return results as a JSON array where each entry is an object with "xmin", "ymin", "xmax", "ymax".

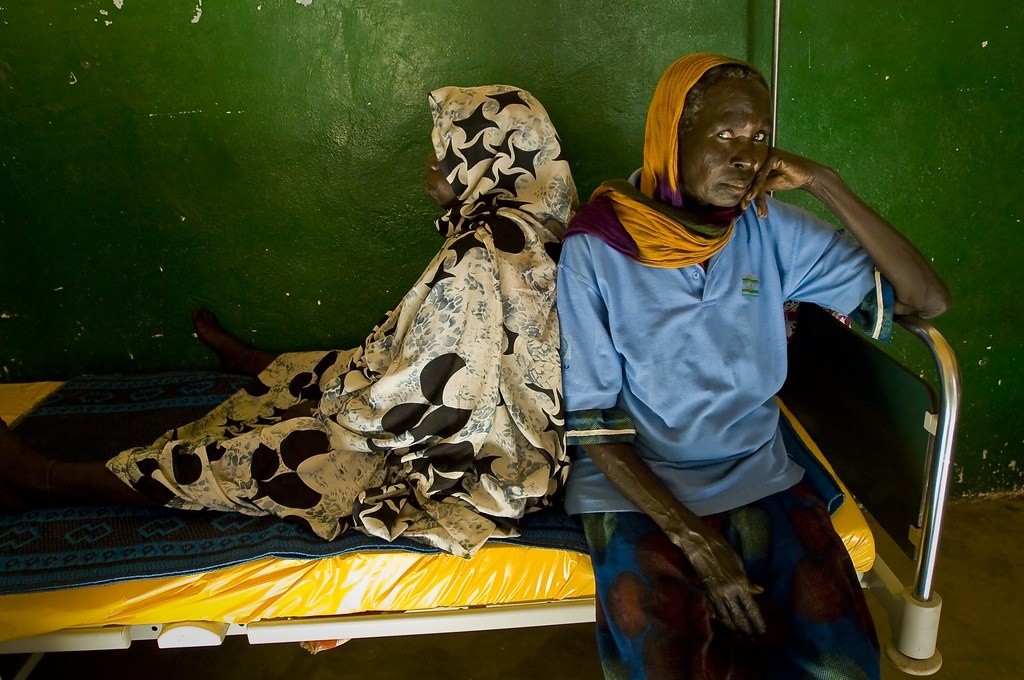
[
  {"xmin": 554, "ymin": 49, "xmax": 952, "ymax": 680},
  {"xmin": 0, "ymin": 84, "xmax": 579, "ymax": 559}
]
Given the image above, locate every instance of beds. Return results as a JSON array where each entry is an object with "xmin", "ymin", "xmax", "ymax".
[{"xmin": 1, "ymin": 301, "xmax": 965, "ymax": 658}]
